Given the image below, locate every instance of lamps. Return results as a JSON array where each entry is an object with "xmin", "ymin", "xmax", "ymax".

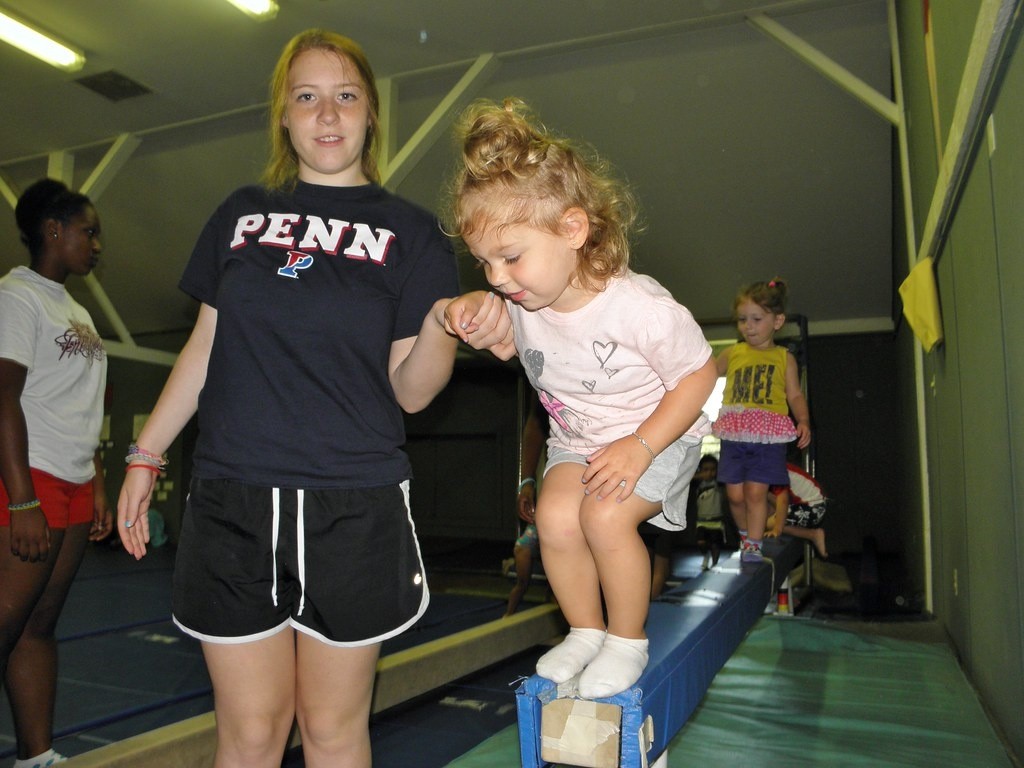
[{"xmin": 0, "ymin": 7, "xmax": 86, "ymax": 74}]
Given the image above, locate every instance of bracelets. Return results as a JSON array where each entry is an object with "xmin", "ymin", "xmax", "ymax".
[
  {"xmin": 632, "ymin": 432, "xmax": 655, "ymax": 465},
  {"xmin": 123, "ymin": 444, "xmax": 167, "ymax": 476},
  {"xmin": 7, "ymin": 499, "xmax": 41, "ymax": 511},
  {"xmin": 517, "ymin": 476, "xmax": 537, "ymax": 495}
]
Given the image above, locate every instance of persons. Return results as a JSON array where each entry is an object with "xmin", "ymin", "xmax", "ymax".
[
  {"xmin": 0, "ymin": 178, "xmax": 113, "ymax": 768},
  {"xmin": 711, "ymin": 275, "xmax": 810, "ymax": 567},
  {"xmin": 114, "ymin": 25, "xmax": 519, "ymax": 768},
  {"xmin": 500, "ymin": 404, "xmax": 727, "ymax": 619},
  {"xmin": 762, "ymin": 462, "xmax": 829, "ymax": 559},
  {"xmin": 441, "ymin": 93, "xmax": 718, "ymax": 700}
]
